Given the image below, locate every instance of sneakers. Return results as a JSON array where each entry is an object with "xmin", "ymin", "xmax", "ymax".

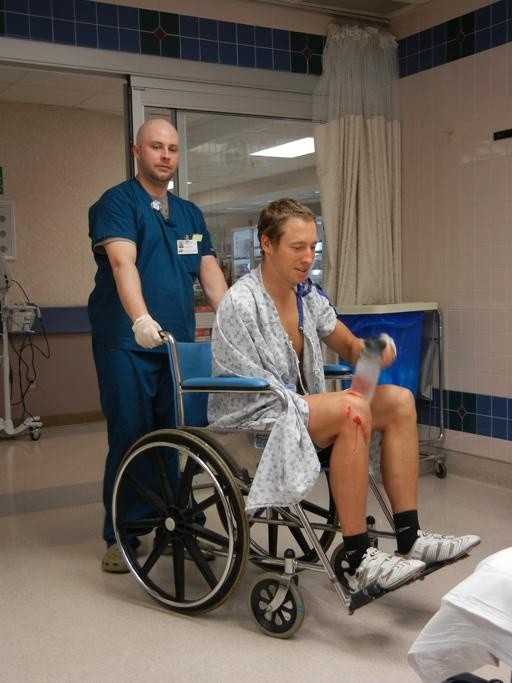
[
  {"xmin": 393, "ymin": 530, "xmax": 481, "ymax": 565},
  {"xmin": 344, "ymin": 548, "xmax": 425, "ymax": 591}
]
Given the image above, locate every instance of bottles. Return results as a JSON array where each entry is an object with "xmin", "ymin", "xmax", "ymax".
[{"xmin": 351, "ymin": 337, "xmax": 385, "ymax": 403}]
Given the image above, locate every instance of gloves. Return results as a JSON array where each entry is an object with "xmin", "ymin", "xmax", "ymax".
[{"xmin": 131, "ymin": 316, "xmax": 167, "ymax": 350}]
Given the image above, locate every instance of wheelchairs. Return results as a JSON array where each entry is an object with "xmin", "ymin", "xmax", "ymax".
[{"xmin": 111, "ymin": 328, "xmax": 470, "ymax": 639}]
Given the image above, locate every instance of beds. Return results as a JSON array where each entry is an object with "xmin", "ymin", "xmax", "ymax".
[{"xmin": 406, "ymin": 538, "xmax": 511, "ymax": 682}]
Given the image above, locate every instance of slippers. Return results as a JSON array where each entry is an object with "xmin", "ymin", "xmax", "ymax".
[{"xmin": 102, "ymin": 543, "xmax": 130, "ymax": 572}]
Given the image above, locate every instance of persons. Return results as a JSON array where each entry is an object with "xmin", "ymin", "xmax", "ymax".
[
  {"xmin": 88, "ymin": 117, "xmax": 229, "ymax": 573},
  {"xmin": 207, "ymin": 198, "xmax": 482, "ymax": 596}
]
